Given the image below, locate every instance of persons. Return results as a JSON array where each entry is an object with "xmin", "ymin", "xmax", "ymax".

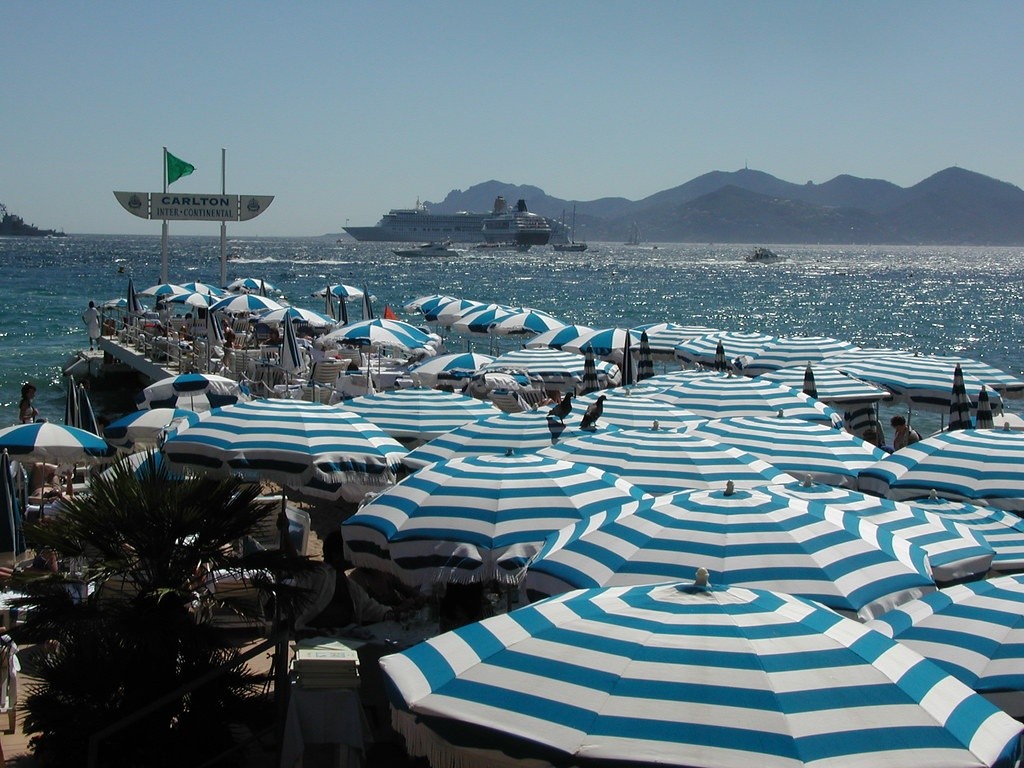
[
  {"xmin": 179, "ymin": 313, "xmax": 193, "ymax": 340},
  {"xmin": 223, "ymin": 330, "xmax": 236, "ymax": 365},
  {"xmin": 220, "ymin": 320, "xmax": 233, "ymax": 333},
  {"xmin": 298, "ymin": 326, "xmax": 315, "ymax": 340},
  {"xmin": 82, "ymin": 300, "xmax": 102, "ymax": 351},
  {"xmin": 890, "ymin": 416, "xmax": 920, "ymax": 451},
  {"xmin": 276, "ymin": 512, "xmax": 394, "ymax": 639},
  {"xmin": 863, "ymin": 429, "xmax": 895, "ymax": 454},
  {"xmin": 0, "ymin": 544, "xmax": 59, "ymax": 587},
  {"xmin": 18, "ymin": 384, "xmax": 38, "ymax": 424},
  {"xmin": 27, "ymin": 462, "xmax": 74, "ymax": 506},
  {"xmin": 163, "ymin": 321, "xmax": 175, "ymax": 337},
  {"xmin": 260, "ymin": 327, "xmax": 284, "ymax": 347},
  {"xmin": 348, "ymin": 567, "xmax": 427, "ymax": 618}
]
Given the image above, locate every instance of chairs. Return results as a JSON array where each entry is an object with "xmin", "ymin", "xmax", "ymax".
[
  {"xmin": 202, "ymin": 495, "xmax": 312, "ymax": 630},
  {"xmin": 488, "ymin": 387, "xmax": 558, "ymax": 414},
  {"xmin": 0, "ymin": 464, "xmax": 96, "ymax": 612},
  {"xmin": 118, "ymin": 316, "xmax": 364, "ymax": 407}
]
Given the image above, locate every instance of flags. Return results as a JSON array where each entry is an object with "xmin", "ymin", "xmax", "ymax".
[{"xmin": 167, "ymin": 152, "xmax": 194, "ymax": 186}]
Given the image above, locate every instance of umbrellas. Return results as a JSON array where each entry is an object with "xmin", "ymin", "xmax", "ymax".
[{"xmin": 0, "ymin": 277, "xmax": 1024, "ymax": 768}]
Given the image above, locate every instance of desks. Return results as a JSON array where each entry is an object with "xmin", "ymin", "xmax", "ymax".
[
  {"xmin": 274, "ymin": 352, "xmax": 415, "ymax": 400},
  {"xmin": 279, "ymin": 661, "xmax": 372, "ymax": 768}
]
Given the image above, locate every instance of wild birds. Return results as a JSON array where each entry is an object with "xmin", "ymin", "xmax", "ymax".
[
  {"xmin": 547, "ymin": 392, "xmax": 575, "ymax": 424},
  {"xmin": 579, "ymin": 395, "xmax": 608, "ymax": 429}
]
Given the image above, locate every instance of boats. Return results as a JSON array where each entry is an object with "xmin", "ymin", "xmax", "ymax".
[
  {"xmin": 341, "ymin": 199, "xmax": 571, "ymax": 243},
  {"xmin": 473, "ymin": 240, "xmax": 531, "ymax": 251},
  {"xmin": 391, "ymin": 240, "xmax": 460, "ymax": 257},
  {"xmin": 481, "ymin": 196, "xmax": 552, "ymax": 245},
  {"xmin": 745, "ymin": 248, "xmax": 786, "ymax": 264},
  {"xmin": 217, "ymin": 253, "xmax": 244, "ymax": 261}
]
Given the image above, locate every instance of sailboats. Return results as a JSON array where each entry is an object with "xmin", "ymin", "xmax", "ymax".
[
  {"xmin": 624, "ymin": 222, "xmax": 641, "ymax": 245},
  {"xmin": 552, "ymin": 204, "xmax": 588, "ymax": 252}
]
